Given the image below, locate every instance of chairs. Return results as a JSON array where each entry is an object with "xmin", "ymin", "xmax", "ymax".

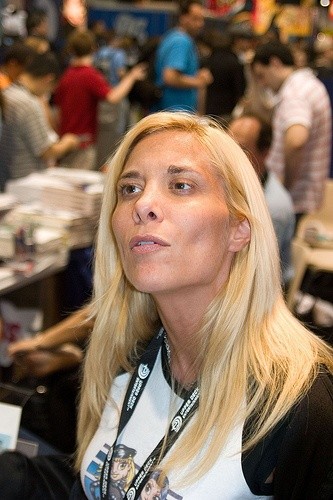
[{"xmin": 287, "ymin": 241, "xmax": 333, "ymax": 325}]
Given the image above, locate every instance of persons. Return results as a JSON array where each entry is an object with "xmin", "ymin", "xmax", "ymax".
[
  {"xmin": 0, "ymin": 1, "xmax": 333, "ymax": 176},
  {"xmin": 251, "ymin": 40, "xmax": 330, "ymax": 238},
  {"xmin": 70, "ymin": 109, "xmax": 332, "ymax": 500},
  {"xmin": 153, "ymin": 3, "xmax": 213, "ymax": 114},
  {"xmin": 222, "ymin": 115, "xmax": 295, "ymax": 292},
  {"xmin": 1, "ymin": 53, "xmax": 78, "ymax": 191}
]
